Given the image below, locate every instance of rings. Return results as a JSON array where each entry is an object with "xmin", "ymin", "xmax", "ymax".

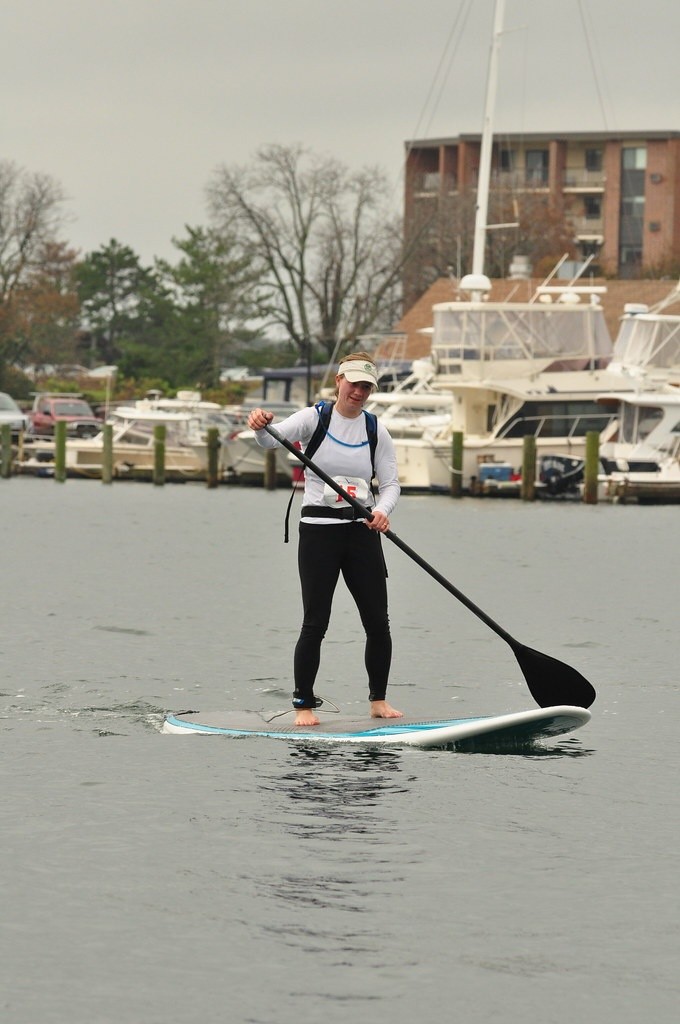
[{"xmin": 383, "ymin": 523, "xmax": 387, "ymax": 528}]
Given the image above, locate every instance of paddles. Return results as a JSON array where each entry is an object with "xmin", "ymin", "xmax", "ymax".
[{"xmin": 263, "ymin": 423, "xmax": 596, "ymax": 709}]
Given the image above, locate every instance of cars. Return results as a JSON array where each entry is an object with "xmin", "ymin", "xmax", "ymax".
[{"xmin": 0, "ymin": 393, "xmax": 33, "ymax": 441}]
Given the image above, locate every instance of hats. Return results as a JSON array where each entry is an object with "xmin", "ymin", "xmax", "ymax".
[{"xmin": 338, "ymin": 359, "xmax": 379, "ymax": 389}]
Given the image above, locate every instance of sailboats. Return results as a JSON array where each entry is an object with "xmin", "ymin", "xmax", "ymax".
[{"xmin": 287, "ymin": 4, "xmax": 680, "ymax": 498}]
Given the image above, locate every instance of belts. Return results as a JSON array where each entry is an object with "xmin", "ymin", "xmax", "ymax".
[{"xmin": 302, "ymin": 507, "xmax": 373, "ymax": 521}]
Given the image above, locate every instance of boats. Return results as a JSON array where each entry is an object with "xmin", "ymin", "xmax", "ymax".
[
  {"xmin": 188, "ymin": 372, "xmax": 317, "ymax": 491},
  {"xmin": 70, "ymin": 407, "xmax": 235, "ymax": 485}
]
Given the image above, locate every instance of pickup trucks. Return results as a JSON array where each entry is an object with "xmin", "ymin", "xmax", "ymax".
[{"xmin": 27, "ymin": 396, "xmax": 101, "ymax": 437}]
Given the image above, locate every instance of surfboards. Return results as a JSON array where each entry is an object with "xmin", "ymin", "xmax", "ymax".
[{"xmin": 164, "ymin": 705, "xmax": 592, "ymax": 747}]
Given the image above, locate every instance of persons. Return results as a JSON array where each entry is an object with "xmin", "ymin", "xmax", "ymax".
[{"xmin": 247, "ymin": 351, "xmax": 403, "ymax": 727}]
